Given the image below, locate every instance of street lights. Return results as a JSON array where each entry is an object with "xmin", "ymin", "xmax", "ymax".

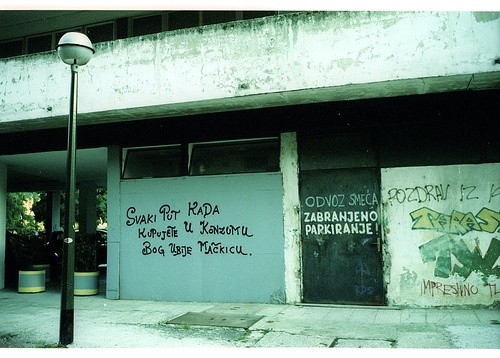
[{"xmin": 56, "ymin": 30, "xmax": 96, "ymax": 346}]
